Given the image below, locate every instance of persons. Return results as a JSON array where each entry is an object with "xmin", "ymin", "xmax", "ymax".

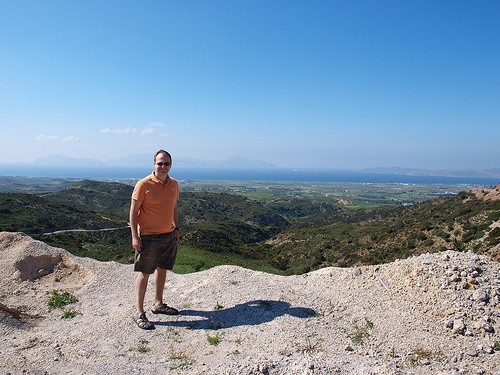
[{"xmin": 128, "ymin": 149, "xmax": 181, "ymax": 330}]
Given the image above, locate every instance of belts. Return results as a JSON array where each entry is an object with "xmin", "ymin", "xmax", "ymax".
[{"xmin": 140, "ymin": 233, "xmax": 175, "ymax": 240}]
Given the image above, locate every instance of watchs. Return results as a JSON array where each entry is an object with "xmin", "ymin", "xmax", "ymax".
[{"xmin": 175, "ymin": 227, "xmax": 180, "ymax": 230}]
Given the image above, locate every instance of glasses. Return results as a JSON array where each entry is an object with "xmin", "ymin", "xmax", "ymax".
[{"xmin": 156, "ymin": 162, "xmax": 170, "ymax": 167}]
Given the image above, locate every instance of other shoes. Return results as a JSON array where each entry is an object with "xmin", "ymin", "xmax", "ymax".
[
  {"xmin": 150, "ymin": 302, "xmax": 179, "ymax": 316},
  {"xmin": 133, "ymin": 312, "xmax": 154, "ymax": 330}
]
